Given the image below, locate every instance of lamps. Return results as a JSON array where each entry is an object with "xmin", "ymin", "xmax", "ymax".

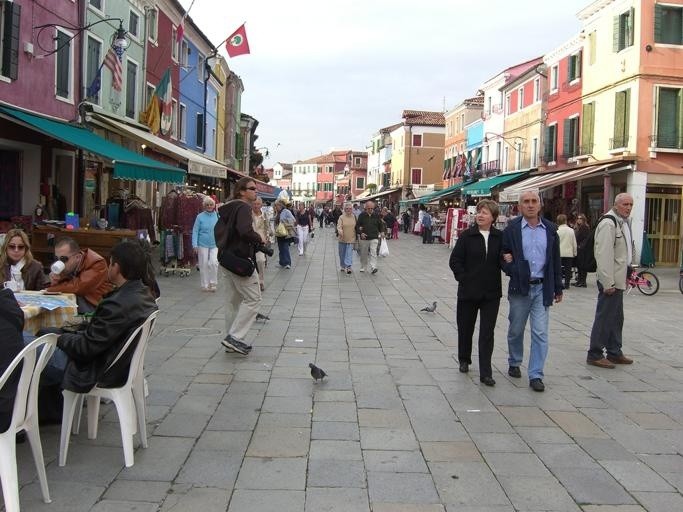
[
  {"xmin": 35, "ymin": 17, "xmax": 132, "ymax": 58},
  {"xmin": 483, "ymin": 131, "xmax": 528, "ymax": 153}
]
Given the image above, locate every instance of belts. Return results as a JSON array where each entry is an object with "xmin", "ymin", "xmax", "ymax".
[{"xmin": 529, "ymin": 279, "xmax": 543, "ymax": 285}]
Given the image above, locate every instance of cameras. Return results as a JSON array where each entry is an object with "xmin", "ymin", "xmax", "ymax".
[{"xmin": 254, "ymin": 242, "xmax": 274, "ymax": 257}]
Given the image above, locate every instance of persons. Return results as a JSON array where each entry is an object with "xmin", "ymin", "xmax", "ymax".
[
  {"xmin": 249, "ymin": 197, "xmax": 440, "ymax": 291},
  {"xmin": 449, "ymin": 199, "xmax": 513, "ymax": 386},
  {"xmin": 0, "ymin": 229, "xmax": 45, "ymax": 291},
  {"xmin": 214, "ymin": 177, "xmax": 268, "ymax": 355},
  {"xmin": 587, "ymin": 193, "xmax": 633, "ymax": 369},
  {"xmin": 498, "ymin": 190, "xmax": 563, "ymax": 392},
  {"xmin": 23, "ymin": 236, "xmax": 158, "ymax": 426},
  {"xmin": 0, "ymin": 258, "xmax": 25, "ymax": 443},
  {"xmin": 554, "ymin": 208, "xmax": 592, "ymax": 291},
  {"xmin": 192, "ymin": 198, "xmax": 221, "ymax": 292}
]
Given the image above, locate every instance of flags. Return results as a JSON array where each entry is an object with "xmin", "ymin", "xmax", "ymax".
[
  {"xmin": 226, "ymin": 24, "xmax": 250, "ymax": 57},
  {"xmin": 139, "ymin": 65, "xmax": 173, "ymax": 136},
  {"xmin": 175, "ymin": 0, "xmax": 195, "ymax": 42},
  {"xmin": 103, "ymin": 35, "xmax": 124, "ymax": 115}
]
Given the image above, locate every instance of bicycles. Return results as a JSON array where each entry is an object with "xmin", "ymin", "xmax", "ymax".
[{"xmin": 624, "ymin": 263, "xmax": 658, "ymax": 297}]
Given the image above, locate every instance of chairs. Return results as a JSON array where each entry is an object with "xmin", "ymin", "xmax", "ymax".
[
  {"xmin": 58, "ymin": 309, "xmax": 162, "ymax": 468},
  {"xmin": 1, "ymin": 333, "xmax": 61, "ymax": 512}
]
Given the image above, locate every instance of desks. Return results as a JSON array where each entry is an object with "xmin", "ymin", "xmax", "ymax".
[{"xmin": 33, "ymin": 225, "xmax": 138, "ymax": 264}]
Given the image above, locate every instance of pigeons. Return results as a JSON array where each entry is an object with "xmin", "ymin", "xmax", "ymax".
[
  {"xmin": 256, "ymin": 312, "xmax": 270, "ymax": 320},
  {"xmin": 420, "ymin": 301, "xmax": 437, "ymax": 315},
  {"xmin": 309, "ymin": 363, "xmax": 328, "ymax": 385}
]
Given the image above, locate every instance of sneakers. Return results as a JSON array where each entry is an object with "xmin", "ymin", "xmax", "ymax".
[
  {"xmin": 560, "ymin": 275, "xmax": 586, "ymax": 289},
  {"xmin": 208, "ymin": 284, "xmax": 217, "ymax": 292},
  {"xmin": 201, "ymin": 287, "xmax": 208, "ymax": 293},
  {"xmin": 285, "ymin": 265, "xmax": 290, "ymax": 270},
  {"xmin": 508, "ymin": 366, "xmax": 521, "ymax": 378},
  {"xmin": 480, "ymin": 376, "xmax": 495, "ymax": 386},
  {"xmin": 221, "ymin": 335, "xmax": 249, "ymax": 355},
  {"xmin": 607, "ymin": 354, "xmax": 633, "ymax": 363},
  {"xmin": 260, "ymin": 284, "xmax": 264, "ymax": 290},
  {"xmin": 530, "ymin": 378, "xmax": 544, "ymax": 392},
  {"xmin": 225, "ymin": 345, "xmax": 252, "ymax": 353},
  {"xmin": 460, "ymin": 363, "xmax": 468, "ymax": 372},
  {"xmin": 341, "ymin": 267, "xmax": 378, "ymax": 274},
  {"xmin": 587, "ymin": 357, "xmax": 614, "ymax": 368}
]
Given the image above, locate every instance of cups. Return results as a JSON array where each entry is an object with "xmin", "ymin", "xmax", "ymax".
[
  {"xmin": 51, "ymin": 261, "xmax": 65, "ymax": 274},
  {"xmin": 3, "ymin": 281, "xmax": 18, "ymax": 292}
]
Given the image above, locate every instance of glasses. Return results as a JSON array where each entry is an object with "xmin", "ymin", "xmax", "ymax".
[
  {"xmin": 55, "ymin": 254, "xmax": 77, "ymax": 262},
  {"xmin": 245, "ymin": 186, "xmax": 257, "ymax": 190},
  {"xmin": 7, "ymin": 244, "xmax": 26, "ymax": 250}
]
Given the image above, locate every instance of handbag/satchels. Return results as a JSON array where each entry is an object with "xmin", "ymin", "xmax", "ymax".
[
  {"xmin": 219, "ymin": 249, "xmax": 254, "ymax": 277},
  {"xmin": 414, "ymin": 223, "xmax": 422, "ymax": 232}
]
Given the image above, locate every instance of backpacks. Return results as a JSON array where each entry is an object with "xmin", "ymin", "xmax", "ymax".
[{"xmin": 578, "ymin": 213, "xmax": 617, "ymax": 272}]
